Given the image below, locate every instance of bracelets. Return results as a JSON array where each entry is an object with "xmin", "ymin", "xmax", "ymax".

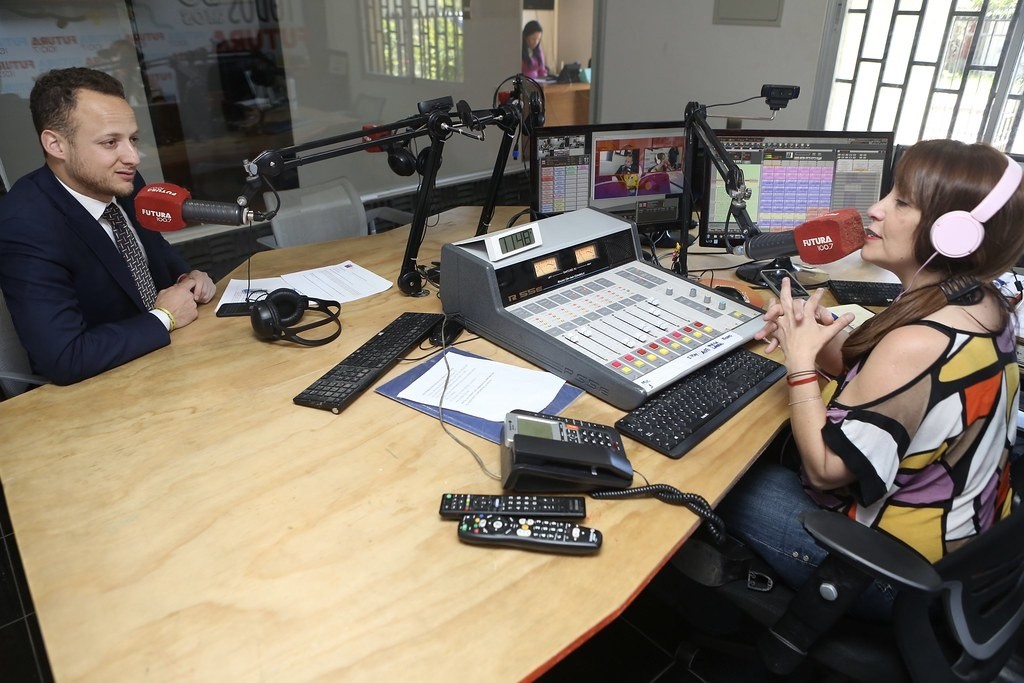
[
  {"xmin": 788, "ymin": 396, "xmax": 822, "ymax": 406},
  {"xmin": 787, "ymin": 370, "xmax": 818, "ymax": 386},
  {"xmin": 152, "ymin": 307, "xmax": 175, "ymax": 332}
]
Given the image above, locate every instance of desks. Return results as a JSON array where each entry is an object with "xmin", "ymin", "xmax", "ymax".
[
  {"xmin": 522, "ymin": 83, "xmax": 590, "ymax": 147},
  {"xmin": 0, "ymin": 204, "xmax": 796, "ymax": 683}
]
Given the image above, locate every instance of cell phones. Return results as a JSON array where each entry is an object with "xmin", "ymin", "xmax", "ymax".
[
  {"xmin": 216, "ymin": 302, "xmax": 253, "ymax": 317},
  {"xmin": 761, "ymin": 268, "xmax": 810, "ymax": 300}
]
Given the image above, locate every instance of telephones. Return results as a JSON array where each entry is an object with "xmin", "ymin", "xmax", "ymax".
[{"xmin": 500, "ymin": 409, "xmax": 634, "ymax": 496}]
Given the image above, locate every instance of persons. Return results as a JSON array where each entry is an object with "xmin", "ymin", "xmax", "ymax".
[
  {"xmin": 648, "ymin": 153, "xmax": 665, "ymax": 172},
  {"xmin": 668, "ymin": 146, "xmax": 679, "ymax": 168},
  {"xmin": 614, "ymin": 155, "xmax": 633, "ymax": 173},
  {"xmin": 521, "ymin": 21, "xmax": 548, "ymax": 78},
  {"xmin": 0, "ymin": 67, "xmax": 216, "ymax": 386},
  {"xmin": 719, "ymin": 139, "xmax": 1024, "ymax": 620}
]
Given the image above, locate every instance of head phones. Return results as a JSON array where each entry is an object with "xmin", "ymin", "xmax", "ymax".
[
  {"xmin": 251, "ymin": 288, "xmax": 341, "ymax": 346},
  {"xmin": 387, "ymin": 142, "xmax": 443, "ymax": 176},
  {"xmin": 929, "ymin": 153, "xmax": 1023, "ymax": 258},
  {"xmin": 493, "ymin": 73, "xmax": 546, "ymax": 135}
]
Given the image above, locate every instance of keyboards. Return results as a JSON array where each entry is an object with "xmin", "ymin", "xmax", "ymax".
[
  {"xmin": 828, "ymin": 280, "xmax": 901, "ymax": 307},
  {"xmin": 293, "ymin": 312, "xmax": 445, "ymax": 415},
  {"xmin": 614, "ymin": 346, "xmax": 788, "ymax": 459}
]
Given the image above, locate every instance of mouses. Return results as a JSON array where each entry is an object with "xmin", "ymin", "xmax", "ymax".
[{"xmin": 429, "ymin": 320, "xmax": 463, "ymax": 346}]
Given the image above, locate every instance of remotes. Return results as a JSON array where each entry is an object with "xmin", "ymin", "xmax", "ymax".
[
  {"xmin": 457, "ymin": 514, "xmax": 603, "ymax": 555},
  {"xmin": 439, "ymin": 493, "xmax": 586, "ymax": 522}
]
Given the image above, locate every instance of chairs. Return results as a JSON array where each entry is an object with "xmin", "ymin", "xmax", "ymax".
[
  {"xmin": 719, "ymin": 503, "xmax": 1024, "ymax": 683},
  {"xmin": 256, "ymin": 177, "xmax": 413, "ymax": 251}
]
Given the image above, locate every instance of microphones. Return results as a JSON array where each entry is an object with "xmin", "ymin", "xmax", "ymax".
[
  {"xmin": 744, "ymin": 208, "xmax": 866, "ymax": 264},
  {"xmin": 134, "ymin": 182, "xmax": 264, "ymax": 232}
]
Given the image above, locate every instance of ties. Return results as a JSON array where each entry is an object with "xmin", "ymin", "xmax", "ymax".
[{"xmin": 102, "ymin": 201, "xmax": 159, "ymax": 311}]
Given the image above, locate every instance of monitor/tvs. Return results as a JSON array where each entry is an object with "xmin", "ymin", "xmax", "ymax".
[
  {"xmin": 529, "ymin": 121, "xmax": 693, "ymax": 234},
  {"xmin": 699, "ymin": 128, "xmax": 895, "ymax": 289},
  {"xmin": 557, "ymin": 63, "xmax": 581, "ymax": 83}
]
